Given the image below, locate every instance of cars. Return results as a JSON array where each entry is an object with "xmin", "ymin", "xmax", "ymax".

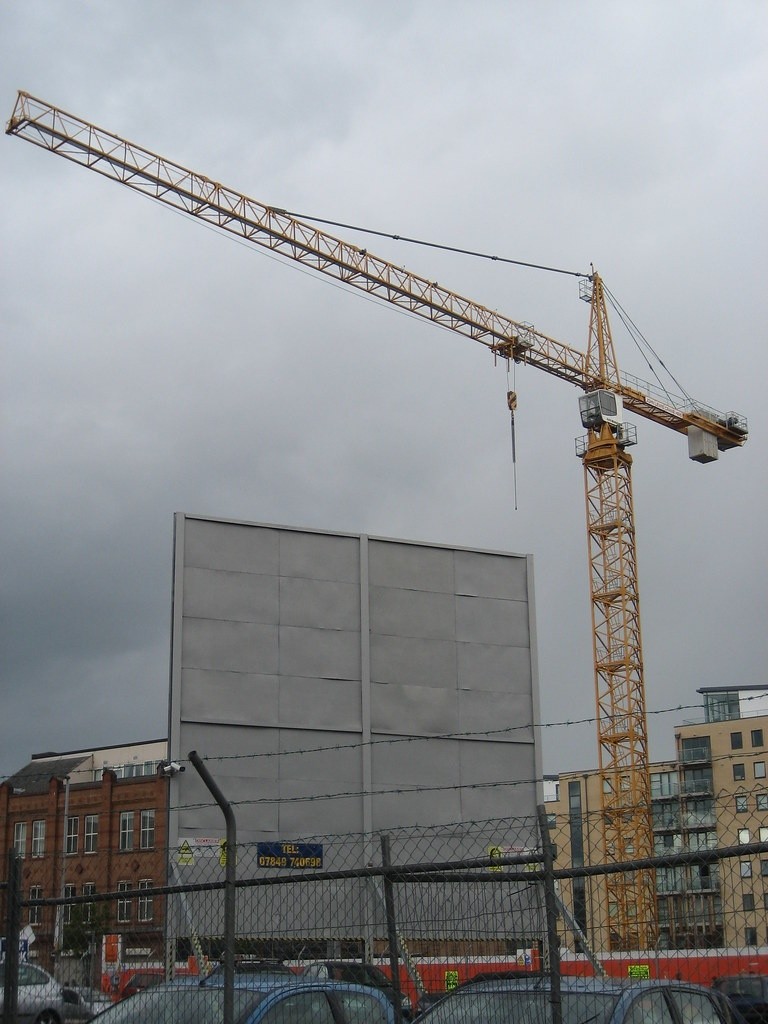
[
  {"xmin": 121, "ymin": 971, "xmax": 166, "ymax": 1000},
  {"xmin": 712, "ymin": 972, "xmax": 768, "ymax": 1024},
  {"xmin": 300, "ymin": 960, "xmax": 412, "ymax": 1017},
  {"xmin": 411, "ymin": 969, "xmax": 566, "ymax": 1020},
  {"xmin": 0, "ymin": 960, "xmax": 66, "ymax": 1024},
  {"xmin": 66, "ymin": 985, "xmax": 114, "ymax": 1014},
  {"xmin": 83, "ymin": 972, "xmax": 396, "ymax": 1024},
  {"xmin": 398, "ymin": 967, "xmax": 747, "ymax": 1024},
  {"xmin": 206, "ymin": 957, "xmax": 297, "ymax": 975}
]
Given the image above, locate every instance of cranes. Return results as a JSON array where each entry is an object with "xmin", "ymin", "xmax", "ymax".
[{"xmin": 2, "ymin": 89, "xmax": 753, "ymax": 945}]
